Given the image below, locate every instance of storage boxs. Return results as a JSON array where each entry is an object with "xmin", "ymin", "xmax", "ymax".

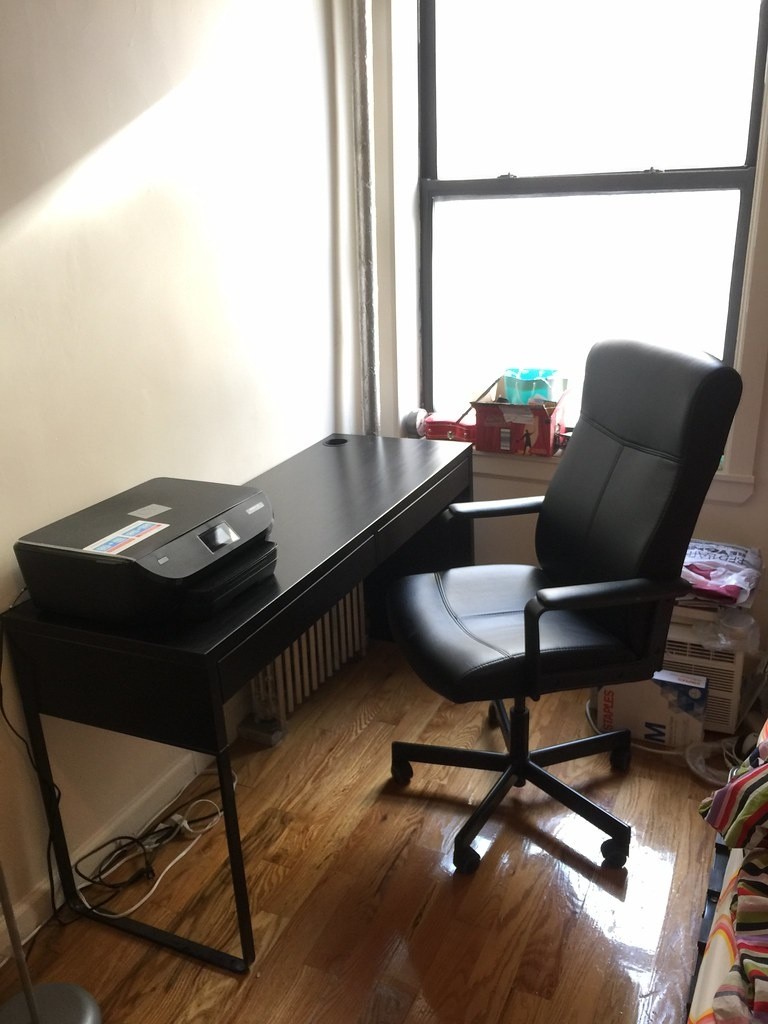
[
  {"xmin": 595, "ymin": 669, "xmax": 709, "ymax": 751},
  {"xmin": 471, "ymin": 379, "xmax": 570, "ymax": 456}
]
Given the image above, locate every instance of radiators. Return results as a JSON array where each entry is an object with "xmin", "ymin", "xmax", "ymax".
[{"xmin": 250, "ymin": 585, "xmax": 366, "ymax": 725}]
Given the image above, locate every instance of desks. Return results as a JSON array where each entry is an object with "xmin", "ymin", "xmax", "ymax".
[{"xmin": 0, "ymin": 433, "xmax": 476, "ymax": 975}]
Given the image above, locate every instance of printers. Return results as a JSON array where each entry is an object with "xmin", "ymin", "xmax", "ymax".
[{"xmin": 11, "ymin": 476, "xmax": 279, "ymax": 628}]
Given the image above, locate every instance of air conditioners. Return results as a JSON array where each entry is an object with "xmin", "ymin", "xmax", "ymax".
[{"xmin": 657, "ymin": 601, "xmax": 766, "ymax": 735}]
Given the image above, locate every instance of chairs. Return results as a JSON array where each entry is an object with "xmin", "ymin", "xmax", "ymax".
[{"xmin": 389, "ymin": 336, "xmax": 744, "ymax": 873}]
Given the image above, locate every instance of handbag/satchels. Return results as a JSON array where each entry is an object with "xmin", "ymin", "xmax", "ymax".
[
  {"xmin": 681, "ymin": 536, "xmax": 761, "ymax": 608},
  {"xmin": 495, "ymin": 367, "xmax": 559, "ymax": 404}
]
